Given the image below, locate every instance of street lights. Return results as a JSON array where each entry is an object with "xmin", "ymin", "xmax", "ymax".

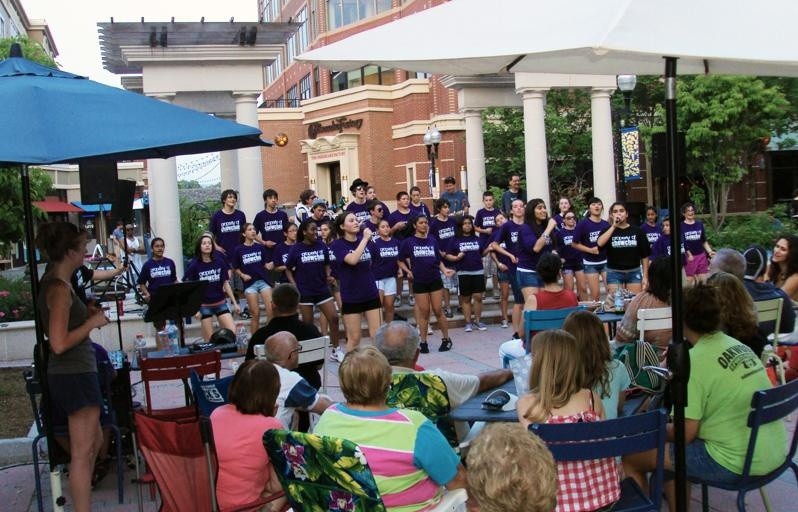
[
  {"xmin": 609, "ymin": 73, "xmax": 637, "ymax": 203},
  {"xmin": 423, "ymin": 124, "xmax": 443, "ymax": 216}
]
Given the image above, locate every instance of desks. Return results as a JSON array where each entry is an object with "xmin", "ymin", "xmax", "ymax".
[
  {"xmin": 449, "ymin": 377, "xmax": 519, "ymax": 457},
  {"xmin": 593, "ymin": 309, "xmax": 624, "ymax": 341}
]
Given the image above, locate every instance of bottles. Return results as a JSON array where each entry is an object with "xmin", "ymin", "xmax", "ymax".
[
  {"xmin": 158, "ymin": 331, "xmax": 167, "ymax": 351},
  {"xmin": 232, "ymin": 362, "xmax": 238, "ymax": 374},
  {"xmin": 235, "ymin": 322, "xmax": 248, "ymax": 354},
  {"xmin": 164, "ymin": 319, "xmax": 182, "ymax": 356},
  {"xmin": 134, "ymin": 334, "xmax": 147, "ymax": 366},
  {"xmin": 117, "ymin": 298, "xmax": 123, "ymax": 317},
  {"xmin": 613, "ymin": 279, "xmax": 624, "ymax": 311}
]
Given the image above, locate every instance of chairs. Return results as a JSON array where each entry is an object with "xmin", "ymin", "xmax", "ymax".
[
  {"xmin": 261, "ymin": 427, "xmax": 387, "ymax": 511},
  {"xmin": 636, "ymin": 307, "xmax": 673, "ymax": 342},
  {"xmin": 528, "ymin": 406, "xmax": 668, "ymax": 511},
  {"xmin": 251, "ymin": 336, "xmax": 329, "ymax": 398},
  {"xmin": 131, "ymin": 411, "xmax": 288, "ymax": 512},
  {"xmin": 682, "ymin": 379, "xmax": 796, "ymax": 511},
  {"xmin": 22, "ymin": 369, "xmax": 131, "ymax": 511},
  {"xmin": 522, "ymin": 305, "xmax": 590, "ymax": 355},
  {"xmin": 134, "ymin": 351, "xmax": 225, "ymax": 418},
  {"xmin": 754, "ymin": 299, "xmax": 782, "ymax": 340}
]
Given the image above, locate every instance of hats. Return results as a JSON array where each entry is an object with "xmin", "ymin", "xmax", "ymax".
[
  {"xmin": 743, "ymin": 247, "xmax": 768, "ymax": 280},
  {"xmin": 350, "ymin": 179, "xmax": 368, "ymax": 191},
  {"xmin": 481, "ymin": 389, "xmax": 518, "ymax": 411},
  {"xmin": 310, "ymin": 199, "xmax": 325, "ymax": 213},
  {"xmin": 211, "ymin": 328, "xmax": 235, "ymax": 344}
]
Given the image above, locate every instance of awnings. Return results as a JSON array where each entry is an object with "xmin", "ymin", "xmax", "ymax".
[
  {"xmin": 35, "ymin": 201, "xmax": 83, "ymax": 212},
  {"xmin": 71, "ymin": 201, "xmax": 112, "ymax": 212},
  {"xmin": 132, "ymin": 198, "xmax": 144, "ymax": 210}
]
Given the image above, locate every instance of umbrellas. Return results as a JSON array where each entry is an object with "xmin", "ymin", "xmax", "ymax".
[
  {"xmin": 293, "ymin": 0, "xmax": 798, "ymax": 512},
  {"xmin": 0, "ymin": 44, "xmax": 274, "ymax": 512}
]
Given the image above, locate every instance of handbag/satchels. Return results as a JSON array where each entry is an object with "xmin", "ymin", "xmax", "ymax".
[
  {"xmin": 604, "ymin": 288, "xmax": 636, "ymax": 311},
  {"xmin": 613, "ymin": 340, "xmax": 661, "ymax": 391}
]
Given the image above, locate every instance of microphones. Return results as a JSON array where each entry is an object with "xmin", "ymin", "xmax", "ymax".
[
  {"xmin": 615, "ymin": 216, "xmax": 621, "ymax": 223},
  {"xmin": 360, "ymin": 223, "xmax": 377, "ymax": 245},
  {"xmin": 547, "ymin": 218, "xmax": 561, "ymax": 232}
]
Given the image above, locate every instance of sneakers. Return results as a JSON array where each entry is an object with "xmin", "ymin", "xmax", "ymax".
[{"xmin": 330, "ymin": 289, "xmax": 520, "ymax": 363}]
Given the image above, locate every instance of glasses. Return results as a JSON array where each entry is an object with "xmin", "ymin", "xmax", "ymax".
[
  {"xmin": 624, "ymin": 387, "xmax": 642, "ymax": 400},
  {"xmin": 127, "ymin": 228, "xmax": 133, "ymax": 231},
  {"xmin": 565, "ymin": 216, "xmax": 575, "ymax": 220},
  {"xmin": 377, "ymin": 208, "xmax": 384, "ymax": 212},
  {"xmin": 357, "ymin": 186, "xmax": 367, "ymax": 190},
  {"xmin": 309, "ymin": 196, "xmax": 316, "ymax": 200},
  {"xmin": 288, "ymin": 345, "xmax": 302, "ymax": 359}
]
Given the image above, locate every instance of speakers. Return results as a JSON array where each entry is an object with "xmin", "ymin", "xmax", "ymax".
[
  {"xmin": 652, "ymin": 131, "xmax": 687, "ymax": 179},
  {"xmin": 79, "ymin": 158, "xmax": 119, "ymax": 204},
  {"xmin": 765, "ymin": 150, "xmax": 798, "ymax": 204},
  {"xmin": 111, "ymin": 180, "xmax": 136, "ymax": 225}
]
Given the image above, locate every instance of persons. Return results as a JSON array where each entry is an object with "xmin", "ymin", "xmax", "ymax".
[
  {"xmin": 183, "ymin": 173, "xmax": 716, "ymax": 363},
  {"xmin": 313, "ymin": 346, "xmax": 466, "ymax": 512},
  {"xmin": 113, "ymin": 222, "xmax": 179, "ymax": 345},
  {"xmin": 466, "ymin": 234, "xmax": 797, "ymax": 512},
  {"xmin": 34, "ymin": 221, "xmax": 128, "ymax": 512},
  {"xmin": 211, "ymin": 331, "xmax": 333, "ymax": 512},
  {"xmin": 376, "ymin": 321, "xmax": 513, "ymax": 409}
]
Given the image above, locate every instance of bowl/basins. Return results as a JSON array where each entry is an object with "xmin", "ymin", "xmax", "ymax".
[{"xmin": 577, "ymin": 301, "xmax": 601, "ymax": 312}]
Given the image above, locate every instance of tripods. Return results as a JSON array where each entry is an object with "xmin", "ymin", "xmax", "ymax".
[{"xmin": 81, "ymin": 203, "xmax": 149, "ymax": 318}]
